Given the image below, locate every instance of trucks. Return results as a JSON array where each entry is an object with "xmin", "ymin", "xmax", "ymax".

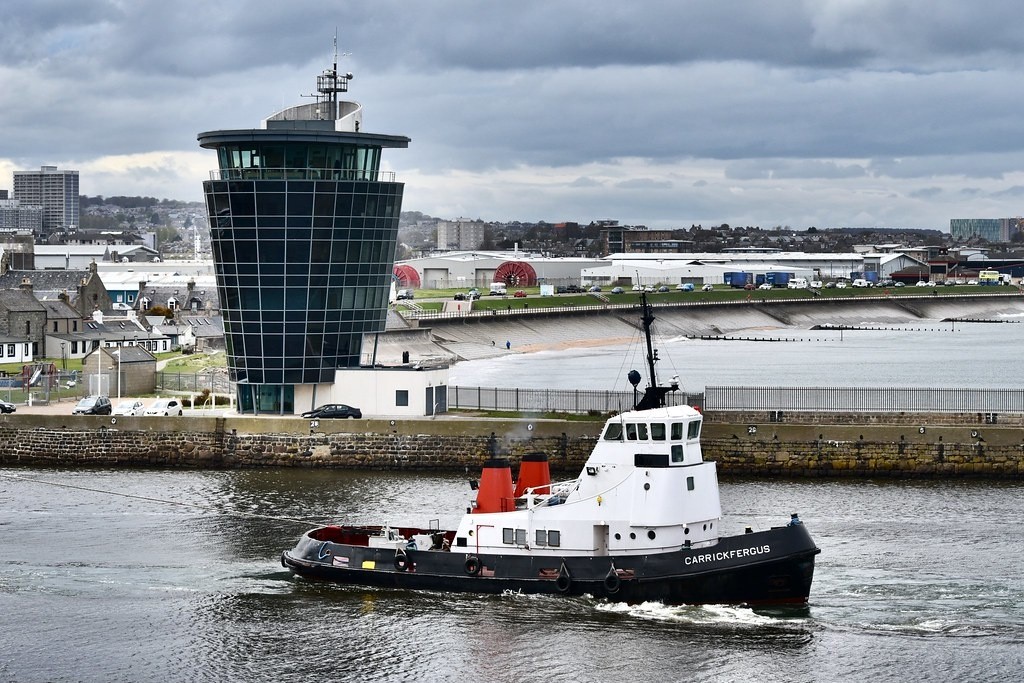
[{"xmin": 489, "ymin": 282, "xmax": 507, "ymax": 296}]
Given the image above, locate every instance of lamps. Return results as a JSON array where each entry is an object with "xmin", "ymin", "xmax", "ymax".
[
  {"xmin": 745, "ymin": 528, "xmax": 752, "ymax": 534},
  {"xmin": 684, "ymin": 540, "xmax": 690, "ymax": 547},
  {"xmin": 471, "ymin": 501, "xmax": 478, "ymax": 508},
  {"xmin": 792, "ymin": 513, "xmax": 798, "ymax": 518},
  {"xmin": 586, "ymin": 467, "xmax": 596, "ymax": 475}
]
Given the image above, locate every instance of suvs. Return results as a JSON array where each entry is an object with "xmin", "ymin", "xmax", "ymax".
[
  {"xmin": 143, "ymin": 398, "xmax": 184, "ymax": 416},
  {"xmin": 71, "ymin": 393, "xmax": 113, "ymax": 415}
]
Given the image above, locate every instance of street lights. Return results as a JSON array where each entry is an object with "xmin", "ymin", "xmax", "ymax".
[
  {"xmin": 175, "ymin": 322, "xmax": 180, "ymax": 344},
  {"xmin": 60, "ymin": 341, "xmax": 66, "ymax": 372},
  {"xmin": 91, "ymin": 346, "xmax": 101, "ymax": 395},
  {"xmin": 193, "ymin": 325, "xmax": 198, "ymax": 355},
  {"xmin": 111, "ymin": 345, "xmax": 121, "ymax": 399},
  {"xmin": 146, "ymin": 327, "xmax": 152, "ymax": 352}
]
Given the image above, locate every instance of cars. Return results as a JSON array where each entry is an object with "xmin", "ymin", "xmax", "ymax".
[
  {"xmin": 701, "ymin": 284, "xmax": 714, "ymax": 292},
  {"xmin": 111, "ymin": 400, "xmax": 147, "ymax": 416},
  {"xmin": 300, "ymin": 403, "xmax": 363, "ymax": 420},
  {"xmin": 454, "ymin": 287, "xmax": 482, "ymax": 301},
  {"xmin": 514, "ymin": 290, "xmax": 528, "ymax": 297},
  {"xmin": 611, "ymin": 287, "xmax": 625, "ymax": 295},
  {"xmin": 556, "ymin": 284, "xmax": 602, "ymax": 294},
  {"xmin": 632, "ymin": 282, "xmax": 695, "ymax": 293},
  {"xmin": 743, "ymin": 271, "xmax": 1024, "ymax": 290},
  {"xmin": 0, "ymin": 399, "xmax": 17, "ymax": 414}
]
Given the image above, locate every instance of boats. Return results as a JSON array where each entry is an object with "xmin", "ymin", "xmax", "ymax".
[{"xmin": 280, "ymin": 284, "xmax": 823, "ymax": 607}]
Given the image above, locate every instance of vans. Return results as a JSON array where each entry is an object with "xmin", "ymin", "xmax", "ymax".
[{"xmin": 396, "ymin": 288, "xmax": 415, "ymax": 300}]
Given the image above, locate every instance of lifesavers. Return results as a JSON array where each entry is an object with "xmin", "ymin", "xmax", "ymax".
[
  {"xmin": 393, "ymin": 554, "xmax": 409, "ymax": 572},
  {"xmin": 462, "ymin": 557, "xmax": 480, "ymax": 575},
  {"xmin": 555, "ymin": 574, "xmax": 571, "ymax": 592},
  {"xmin": 603, "ymin": 574, "xmax": 620, "ymax": 593}
]
